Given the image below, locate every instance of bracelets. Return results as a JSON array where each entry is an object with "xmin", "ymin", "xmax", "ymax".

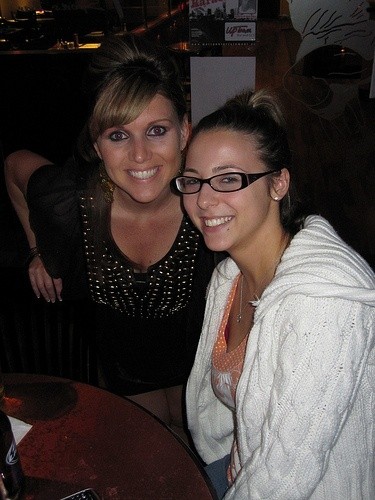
[{"xmin": 29, "ymin": 247, "xmax": 41, "ymax": 258}]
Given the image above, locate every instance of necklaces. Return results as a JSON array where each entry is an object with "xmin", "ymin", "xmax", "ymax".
[{"xmin": 236, "ymin": 230, "xmax": 290, "ymax": 323}]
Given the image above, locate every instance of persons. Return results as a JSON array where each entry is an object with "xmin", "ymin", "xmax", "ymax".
[
  {"xmin": 172, "ymin": 86, "xmax": 375, "ymax": 500},
  {"xmin": 239, "ymin": 0, "xmax": 256, "ymax": 13},
  {"xmin": 3, "ymin": 36, "xmax": 233, "ymax": 467}
]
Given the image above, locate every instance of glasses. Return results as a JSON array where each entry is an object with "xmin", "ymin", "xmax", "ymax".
[{"xmin": 169, "ymin": 169, "xmax": 280, "ymax": 194}]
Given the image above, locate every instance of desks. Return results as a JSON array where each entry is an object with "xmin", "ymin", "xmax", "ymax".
[{"xmin": 1, "ymin": 372, "xmax": 218, "ymax": 498}]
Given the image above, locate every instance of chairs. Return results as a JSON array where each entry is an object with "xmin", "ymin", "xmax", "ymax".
[{"xmin": 1, "ymin": 266, "xmax": 100, "ymax": 387}]
